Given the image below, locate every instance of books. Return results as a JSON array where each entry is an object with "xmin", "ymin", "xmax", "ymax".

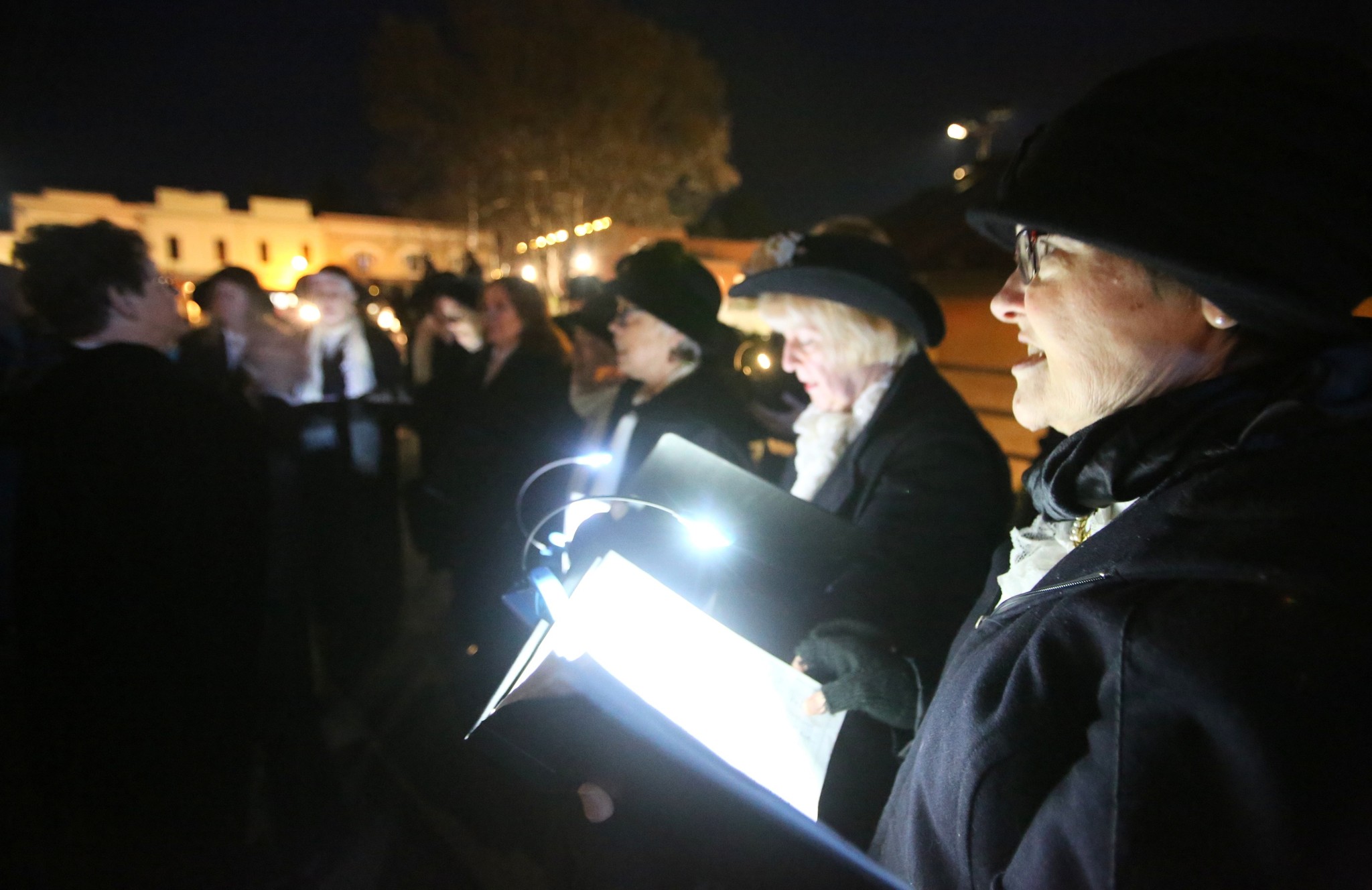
[{"xmin": 466, "ymin": 551, "xmax": 906, "ymax": 890}]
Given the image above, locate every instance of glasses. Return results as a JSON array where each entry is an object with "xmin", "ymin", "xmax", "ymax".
[{"xmin": 1013, "ymin": 226, "xmax": 1099, "ymax": 285}]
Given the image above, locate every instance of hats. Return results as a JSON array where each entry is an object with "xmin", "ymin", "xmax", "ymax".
[
  {"xmin": 961, "ymin": 106, "xmax": 1285, "ymax": 323},
  {"xmin": 728, "ymin": 233, "xmax": 947, "ymax": 349},
  {"xmin": 294, "ymin": 263, "xmax": 369, "ymax": 311},
  {"xmin": 608, "ymin": 245, "xmax": 745, "ymax": 354},
  {"xmin": 190, "ymin": 268, "xmax": 263, "ymax": 319}
]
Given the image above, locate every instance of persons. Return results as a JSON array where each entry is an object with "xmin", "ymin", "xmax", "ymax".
[
  {"xmin": 579, "ymin": 20, "xmax": 1372, "ymax": 890},
  {"xmin": 0, "ymin": 223, "xmax": 1015, "ymax": 890}
]
